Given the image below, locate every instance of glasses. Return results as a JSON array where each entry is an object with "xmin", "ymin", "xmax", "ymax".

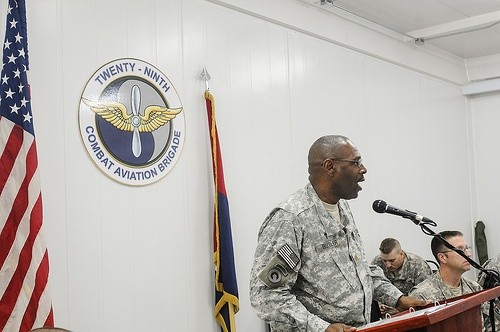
[
  {"xmin": 321, "ymin": 157, "xmax": 362, "ymax": 168},
  {"xmin": 437, "ymin": 246, "xmax": 469, "ymax": 255}
]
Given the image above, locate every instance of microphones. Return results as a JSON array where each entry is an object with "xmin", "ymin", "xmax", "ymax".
[{"xmin": 372, "ymin": 200, "xmax": 433, "ymax": 223}]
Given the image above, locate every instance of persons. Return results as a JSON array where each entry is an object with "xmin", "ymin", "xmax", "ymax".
[
  {"xmin": 370, "ymin": 238, "xmax": 433, "ymax": 318},
  {"xmin": 408, "ymin": 231, "xmax": 500, "ymax": 332},
  {"xmin": 249, "ymin": 134, "xmax": 432, "ymax": 332}
]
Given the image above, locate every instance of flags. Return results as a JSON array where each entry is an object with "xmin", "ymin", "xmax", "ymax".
[
  {"xmin": 0, "ymin": 0, "xmax": 54, "ymax": 332},
  {"xmin": 204, "ymin": 90, "xmax": 240, "ymax": 332}
]
